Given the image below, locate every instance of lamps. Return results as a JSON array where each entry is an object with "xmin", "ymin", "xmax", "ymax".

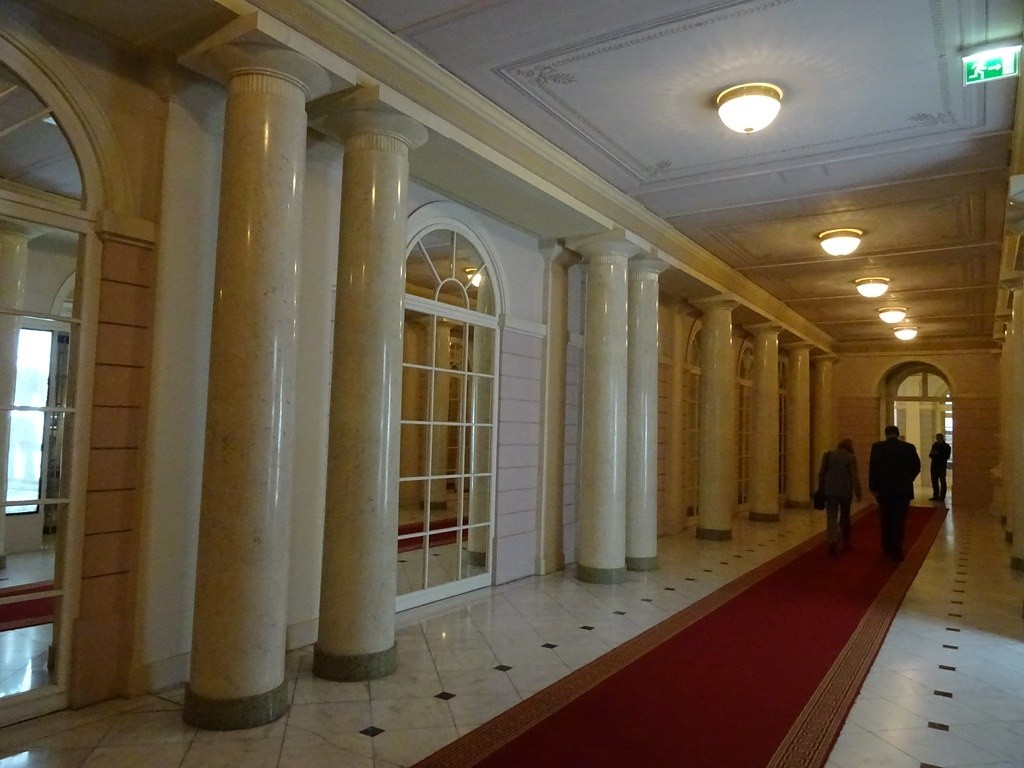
[
  {"xmin": 854, "ymin": 277, "xmax": 888, "ymax": 298},
  {"xmin": 818, "ymin": 228, "xmax": 864, "ymax": 258},
  {"xmin": 893, "ymin": 325, "xmax": 918, "ymax": 340},
  {"xmin": 877, "ymin": 306, "xmax": 907, "ymax": 324},
  {"xmin": 716, "ymin": 82, "xmax": 784, "ymax": 135},
  {"xmin": 466, "ymin": 268, "xmax": 482, "ymax": 288}
]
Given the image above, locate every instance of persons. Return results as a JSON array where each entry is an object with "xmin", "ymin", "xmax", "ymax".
[
  {"xmin": 818, "ymin": 437, "xmax": 862, "ymax": 552},
  {"xmin": 868, "ymin": 425, "xmax": 921, "ymax": 564},
  {"xmin": 928, "ymin": 433, "xmax": 951, "ymax": 502}
]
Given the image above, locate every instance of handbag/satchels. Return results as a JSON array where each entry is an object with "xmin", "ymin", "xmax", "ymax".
[{"xmin": 813, "ymin": 482, "xmax": 826, "ymax": 510}]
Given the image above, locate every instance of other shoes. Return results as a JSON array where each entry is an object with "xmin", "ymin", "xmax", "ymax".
[{"xmin": 929, "ymin": 497, "xmax": 944, "ymax": 502}]
